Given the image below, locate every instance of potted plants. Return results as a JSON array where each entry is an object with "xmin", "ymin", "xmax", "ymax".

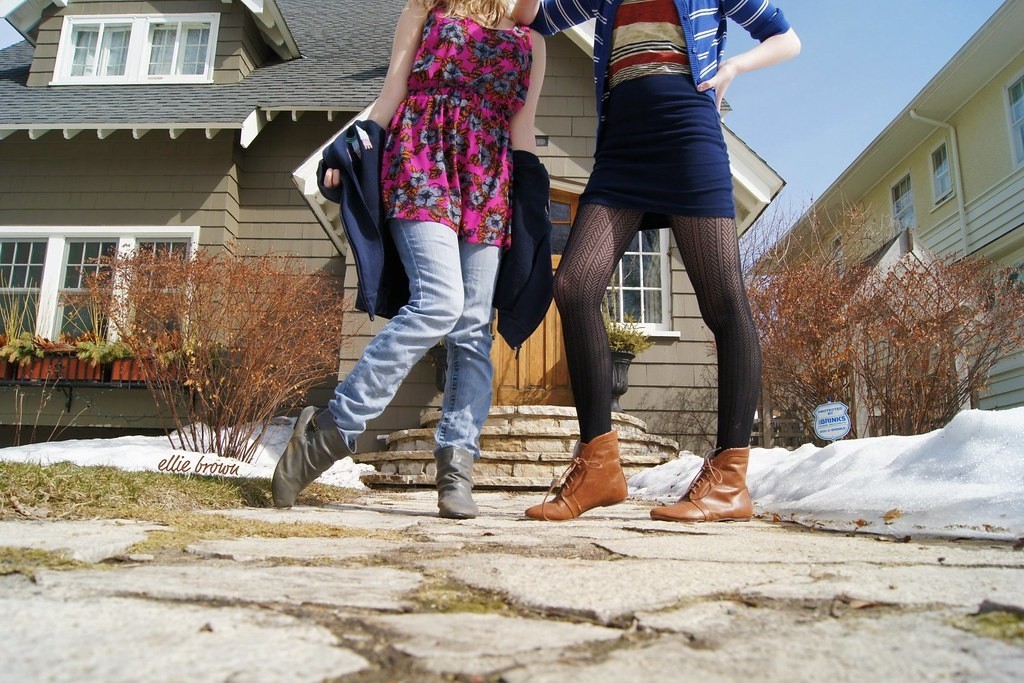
[
  {"xmin": 110, "ymin": 337, "xmax": 177, "ymax": 385},
  {"xmin": 600, "ymin": 274, "xmax": 655, "ymax": 413},
  {"xmin": 0, "ymin": 331, "xmax": 100, "ymax": 380}
]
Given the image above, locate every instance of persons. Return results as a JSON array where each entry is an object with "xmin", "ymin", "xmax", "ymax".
[
  {"xmin": 269, "ymin": 0, "xmax": 547, "ymax": 520},
  {"xmin": 524, "ymin": 0, "xmax": 803, "ymax": 522}
]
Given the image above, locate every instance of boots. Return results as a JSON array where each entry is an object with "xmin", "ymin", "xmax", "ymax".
[
  {"xmin": 271, "ymin": 406, "xmax": 357, "ymax": 511},
  {"xmin": 433, "ymin": 446, "xmax": 480, "ymax": 519},
  {"xmin": 651, "ymin": 444, "xmax": 754, "ymax": 521},
  {"xmin": 524, "ymin": 429, "xmax": 628, "ymax": 520}
]
